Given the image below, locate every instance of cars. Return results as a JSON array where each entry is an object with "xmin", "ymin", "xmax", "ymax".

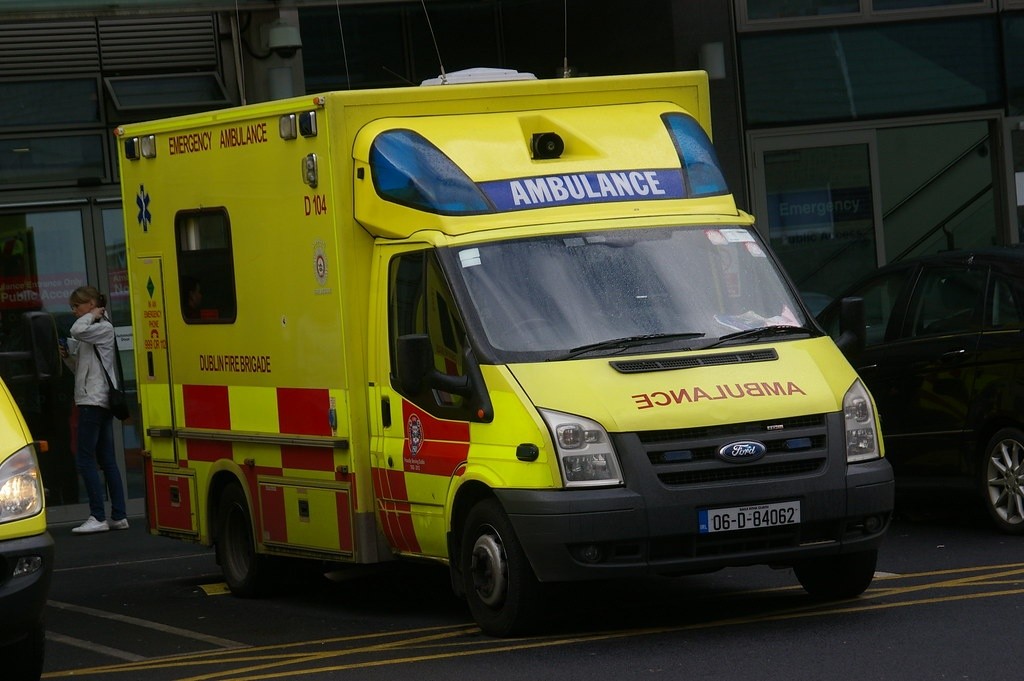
[{"xmin": 816, "ymin": 248, "xmax": 1024, "ymax": 535}]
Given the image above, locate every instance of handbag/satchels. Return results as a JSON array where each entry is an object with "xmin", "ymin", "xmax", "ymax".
[{"xmin": 107, "ymin": 388, "xmax": 130, "ymax": 421}]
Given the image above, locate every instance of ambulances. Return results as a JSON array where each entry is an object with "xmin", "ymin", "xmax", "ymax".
[
  {"xmin": 0, "ymin": 312, "xmax": 67, "ymax": 681},
  {"xmin": 112, "ymin": 67, "xmax": 894, "ymax": 637}
]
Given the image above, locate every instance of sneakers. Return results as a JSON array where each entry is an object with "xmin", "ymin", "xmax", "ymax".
[
  {"xmin": 108, "ymin": 518, "xmax": 129, "ymax": 529},
  {"xmin": 71, "ymin": 515, "xmax": 109, "ymax": 533}
]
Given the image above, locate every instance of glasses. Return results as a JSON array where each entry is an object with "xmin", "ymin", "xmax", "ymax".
[{"xmin": 70, "ymin": 303, "xmax": 80, "ymax": 310}]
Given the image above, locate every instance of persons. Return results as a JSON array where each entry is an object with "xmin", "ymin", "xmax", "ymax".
[{"xmin": 55, "ymin": 284, "xmax": 129, "ymax": 531}]
